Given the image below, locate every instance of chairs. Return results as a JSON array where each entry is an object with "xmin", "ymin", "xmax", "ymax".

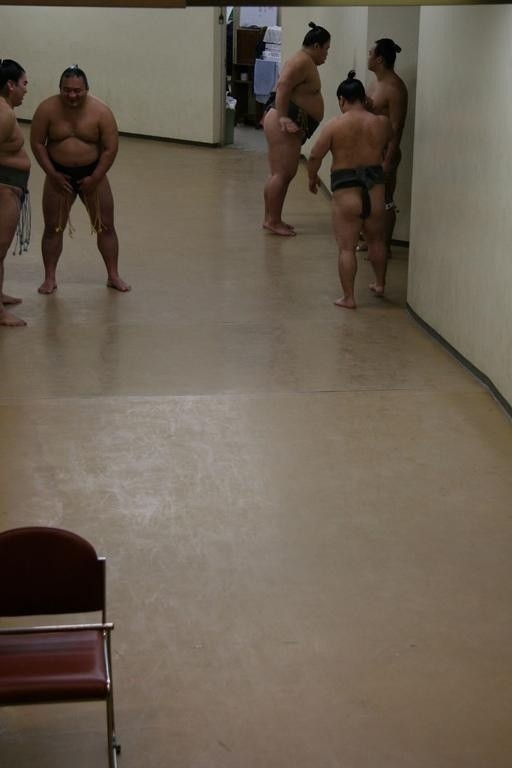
[{"xmin": 0, "ymin": 525, "xmax": 120, "ymax": 768}]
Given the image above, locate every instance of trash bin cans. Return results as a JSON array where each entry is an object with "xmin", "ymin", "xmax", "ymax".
[{"xmin": 224, "ymin": 99, "xmax": 237, "ymax": 144}]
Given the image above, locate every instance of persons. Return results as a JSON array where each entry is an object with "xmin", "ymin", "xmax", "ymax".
[
  {"xmin": 30, "ymin": 66, "xmax": 129, "ymax": 294},
  {"xmin": 357, "ymin": 39, "xmax": 409, "ymax": 260},
  {"xmin": 264, "ymin": 21, "xmax": 331, "ymax": 237},
  {"xmin": 307, "ymin": 71, "xmax": 395, "ymax": 308},
  {"xmin": 0, "ymin": 57, "xmax": 32, "ymax": 326}
]
